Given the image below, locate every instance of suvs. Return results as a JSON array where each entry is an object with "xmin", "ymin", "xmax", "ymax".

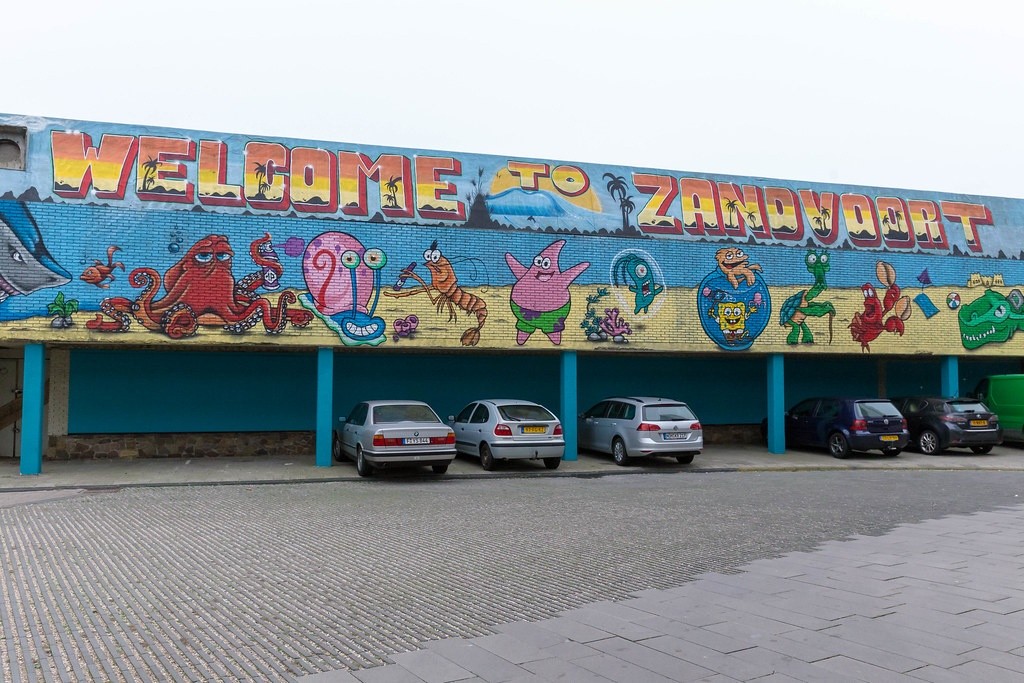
[
  {"xmin": 897, "ymin": 397, "xmax": 1004, "ymax": 457},
  {"xmin": 576, "ymin": 397, "xmax": 704, "ymax": 463},
  {"xmin": 763, "ymin": 398, "xmax": 908, "ymax": 458}
]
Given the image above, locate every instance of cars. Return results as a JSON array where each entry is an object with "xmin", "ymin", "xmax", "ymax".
[
  {"xmin": 333, "ymin": 400, "xmax": 457, "ymax": 477},
  {"xmin": 446, "ymin": 398, "xmax": 564, "ymax": 471}
]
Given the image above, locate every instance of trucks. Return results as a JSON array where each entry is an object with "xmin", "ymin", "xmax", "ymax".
[{"xmin": 965, "ymin": 375, "xmax": 1024, "ymax": 444}]
